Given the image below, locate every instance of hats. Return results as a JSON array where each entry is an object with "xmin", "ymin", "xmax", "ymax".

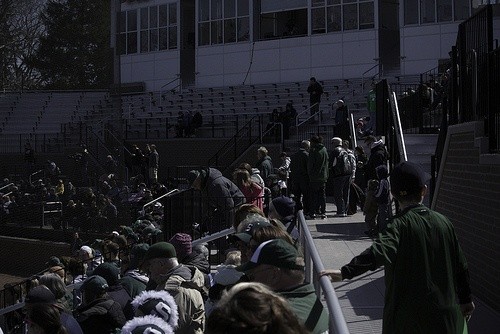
[
  {"xmin": 332, "ymin": 137, "xmax": 342, "ymax": 147},
  {"xmin": 186, "ymin": 171, "xmax": 198, "ymax": 188},
  {"xmin": 79, "ymin": 246, "xmax": 93, "ymax": 256},
  {"xmin": 24, "ymin": 284, "xmax": 55, "ymax": 303},
  {"xmin": 77, "ymin": 275, "xmax": 108, "ymax": 293},
  {"xmin": 138, "ymin": 242, "xmax": 176, "ymax": 266},
  {"xmin": 390, "ymin": 163, "xmax": 424, "ymax": 197},
  {"xmin": 46, "ymin": 257, "xmax": 60, "ymax": 266},
  {"xmin": 271, "ymin": 196, "xmax": 296, "ymax": 218},
  {"xmin": 235, "ymin": 239, "xmax": 304, "ymax": 273},
  {"xmin": 169, "ymin": 233, "xmax": 191, "ymax": 259},
  {"xmin": 120, "ymin": 314, "xmax": 174, "ymax": 334},
  {"xmin": 131, "ymin": 290, "xmax": 178, "ymax": 329}
]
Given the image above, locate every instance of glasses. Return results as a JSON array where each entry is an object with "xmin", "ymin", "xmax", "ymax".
[{"xmin": 242, "ymin": 265, "xmax": 281, "ymax": 282}]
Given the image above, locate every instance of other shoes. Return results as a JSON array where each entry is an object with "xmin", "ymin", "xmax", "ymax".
[
  {"xmin": 305, "ymin": 213, "xmax": 316, "ymax": 220},
  {"xmin": 321, "ymin": 215, "xmax": 327, "ymax": 219},
  {"xmin": 336, "ymin": 211, "xmax": 347, "ymax": 217},
  {"xmin": 364, "ymin": 228, "xmax": 373, "ymax": 236}
]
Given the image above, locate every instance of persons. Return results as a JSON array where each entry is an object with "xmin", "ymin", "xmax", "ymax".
[
  {"xmin": 366, "ymin": 81, "xmax": 377, "ymax": 135},
  {"xmin": 0, "ymin": 198, "xmax": 331, "ymax": 334},
  {"xmin": 306, "ymin": 77, "xmax": 323, "ymax": 125},
  {"xmin": 188, "ymin": 145, "xmax": 291, "ymax": 235},
  {"xmin": 397, "ymin": 63, "xmax": 461, "ymax": 124},
  {"xmin": 319, "ymin": 161, "xmax": 476, "ymax": 334},
  {"xmin": 333, "ymin": 99, "xmax": 349, "ymax": 140},
  {"xmin": 0, "ymin": 138, "xmax": 179, "ymax": 232},
  {"xmin": 290, "ymin": 134, "xmax": 390, "ymax": 236},
  {"xmin": 173, "ymin": 110, "xmax": 203, "ymax": 139},
  {"xmin": 265, "ymin": 103, "xmax": 298, "ymax": 143}
]
